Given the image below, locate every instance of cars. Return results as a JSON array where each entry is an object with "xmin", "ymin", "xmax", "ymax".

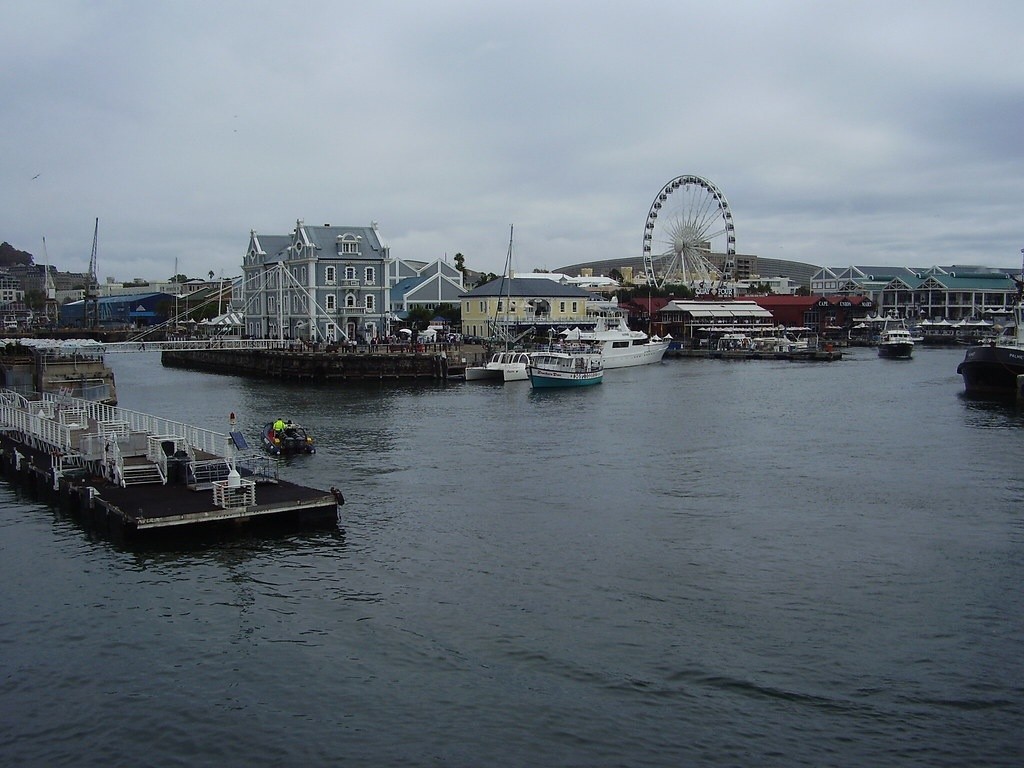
[
  {"xmin": 389, "ymin": 340, "xmax": 420, "ymax": 352},
  {"xmin": 326, "ymin": 341, "xmax": 353, "ymax": 353},
  {"xmin": 465, "ymin": 335, "xmax": 485, "ymax": 344}
]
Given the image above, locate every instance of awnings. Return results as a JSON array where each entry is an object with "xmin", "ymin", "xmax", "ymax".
[{"xmin": 206, "ymin": 312, "xmax": 246, "ymax": 326}]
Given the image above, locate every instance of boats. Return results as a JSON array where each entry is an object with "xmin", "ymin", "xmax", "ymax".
[
  {"xmin": 261, "ymin": 421, "xmax": 317, "ymax": 457},
  {"xmin": 878, "ymin": 328, "xmax": 914, "ymax": 359},
  {"xmin": 20, "ymin": 338, "xmax": 107, "ymax": 352},
  {"xmin": 555, "ymin": 317, "xmax": 671, "ymax": 368},
  {"xmin": 957, "ymin": 249, "xmax": 1024, "ymax": 395},
  {"xmin": 525, "ymin": 352, "xmax": 605, "ymax": 388},
  {"xmin": 31, "ymin": 353, "xmax": 118, "ymax": 418}
]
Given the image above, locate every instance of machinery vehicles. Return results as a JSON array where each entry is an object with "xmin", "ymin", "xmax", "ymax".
[
  {"xmin": 83, "ymin": 217, "xmax": 103, "ymax": 297},
  {"xmin": 42, "ymin": 236, "xmax": 56, "ymax": 300}
]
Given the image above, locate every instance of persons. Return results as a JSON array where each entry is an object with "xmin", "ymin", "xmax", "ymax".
[
  {"xmin": 370, "ymin": 338, "xmax": 379, "ymax": 354},
  {"xmin": 138, "ymin": 336, "xmax": 146, "ymax": 351},
  {"xmin": 273, "ymin": 418, "xmax": 287, "ymax": 439},
  {"xmin": 729, "ymin": 338, "xmax": 757, "ymax": 350},
  {"xmin": 342, "ymin": 337, "xmax": 358, "ymax": 354},
  {"xmin": 381, "ymin": 334, "xmax": 462, "ymax": 353}
]
